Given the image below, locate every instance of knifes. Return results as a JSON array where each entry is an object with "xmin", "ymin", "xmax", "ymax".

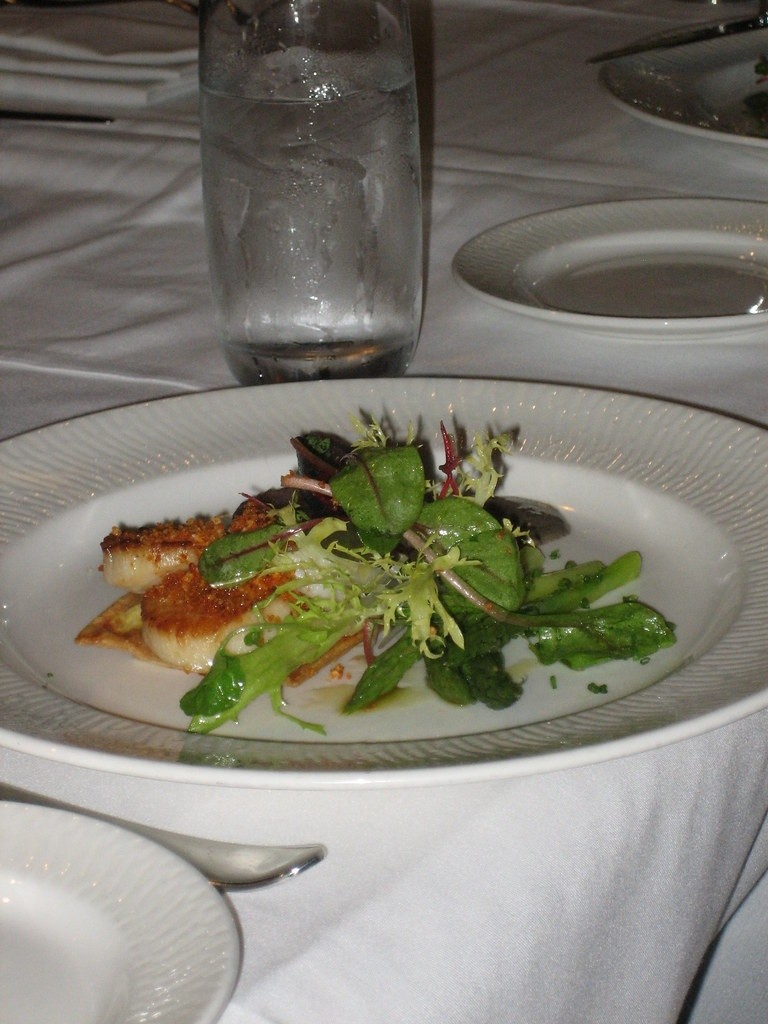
[{"xmin": 586, "ymin": 17, "xmax": 768, "ymax": 65}]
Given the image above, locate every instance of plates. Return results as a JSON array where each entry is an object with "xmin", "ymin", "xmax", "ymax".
[
  {"xmin": 0, "ymin": 382, "xmax": 767, "ymax": 787},
  {"xmin": 451, "ymin": 198, "xmax": 768, "ymax": 340},
  {"xmin": 596, "ymin": 16, "xmax": 768, "ymax": 149},
  {"xmin": 0, "ymin": 800, "xmax": 238, "ymax": 1024}
]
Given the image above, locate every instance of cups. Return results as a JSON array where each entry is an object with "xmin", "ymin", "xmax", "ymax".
[{"xmin": 200, "ymin": 1, "xmax": 419, "ymax": 382}]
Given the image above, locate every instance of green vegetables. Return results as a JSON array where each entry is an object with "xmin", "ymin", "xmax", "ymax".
[{"xmin": 180, "ymin": 414, "xmax": 677, "ymax": 734}]
